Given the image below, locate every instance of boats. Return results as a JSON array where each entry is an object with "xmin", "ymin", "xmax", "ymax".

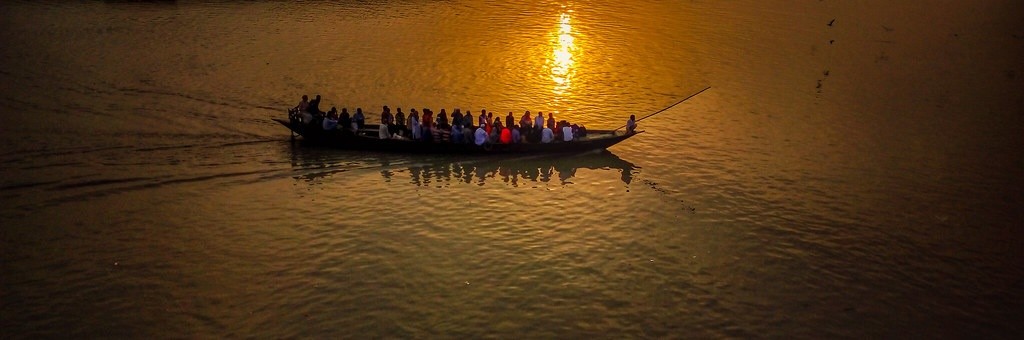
[{"xmin": 272, "ymin": 117, "xmax": 646, "ymax": 155}]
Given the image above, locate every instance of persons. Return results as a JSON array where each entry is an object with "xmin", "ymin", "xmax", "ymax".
[
  {"xmin": 627, "ymin": 115, "xmax": 637, "ymax": 132},
  {"xmin": 297, "ymin": 94, "xmax": 366, "ymax": 136},
  {"xmin": 378, "ymin": 105, "xmax": 586, "ymax": 145}
]
